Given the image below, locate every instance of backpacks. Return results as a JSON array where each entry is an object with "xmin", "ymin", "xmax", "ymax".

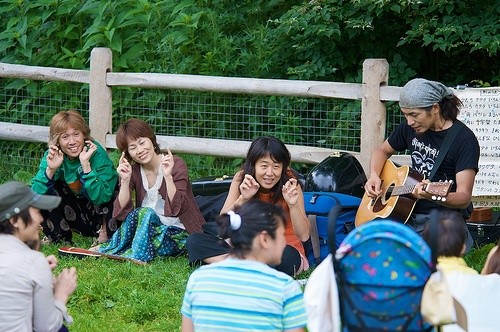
[{"xmin": 303, "ymin": 190, "xmax": 363, "ymax": 267}]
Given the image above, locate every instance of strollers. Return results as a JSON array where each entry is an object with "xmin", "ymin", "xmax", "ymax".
[{"xmin": 327, "ymin": 204, "xmax": 440, "ymax": 332}]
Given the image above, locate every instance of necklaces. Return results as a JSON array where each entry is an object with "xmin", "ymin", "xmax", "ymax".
[{"xmin": 427, "ymin": 117, "xmax": 446, "ymax": 146}]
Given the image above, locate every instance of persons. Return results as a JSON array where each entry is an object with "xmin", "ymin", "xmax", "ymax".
[
  {"xmin": 363, "ymin": 78, "xmax": 481, "ymax": 253},
  {"xmin": 186, "ymin": 136, "xmax": 312, "ymax": 278},
  {"xmin": 97, "ymin": 118, "xmax": 206, "ymax": 263},
  {"xmin": 0, "ymin": 182, "xmax": 79, "ymax": 332},
  {"xmin": 422, "ymin": 208, "xmax": 481, "ymax": 276},
  {"xmin": 180, "ymin": 200, "xmax": 307, "ymax": 332},
  {"xmin": 28, "ymin": 110, "xmax": 123, "ymax": 247}
]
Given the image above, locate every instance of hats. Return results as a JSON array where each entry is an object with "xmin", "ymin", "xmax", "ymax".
[
  {"xmin": 421, "ymin": 272, "xmax": 468, "ymax": 331},
  {"xmin": 0, "ymin": 181, "xmax": 61, "ymax": 222}
]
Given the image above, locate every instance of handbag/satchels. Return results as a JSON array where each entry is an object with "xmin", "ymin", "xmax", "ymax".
[
  {"xmin": 191, "ymin": 175, "xmax": 236, "ymax": 197},
  {"xmin": 305, "ymin": 152, "xmax": 368, "ymax": 199}
]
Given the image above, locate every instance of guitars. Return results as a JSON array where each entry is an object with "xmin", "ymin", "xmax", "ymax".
[{"xmin": 354, "ymin": 159, "xmax": 453, "ymax": 228}]
